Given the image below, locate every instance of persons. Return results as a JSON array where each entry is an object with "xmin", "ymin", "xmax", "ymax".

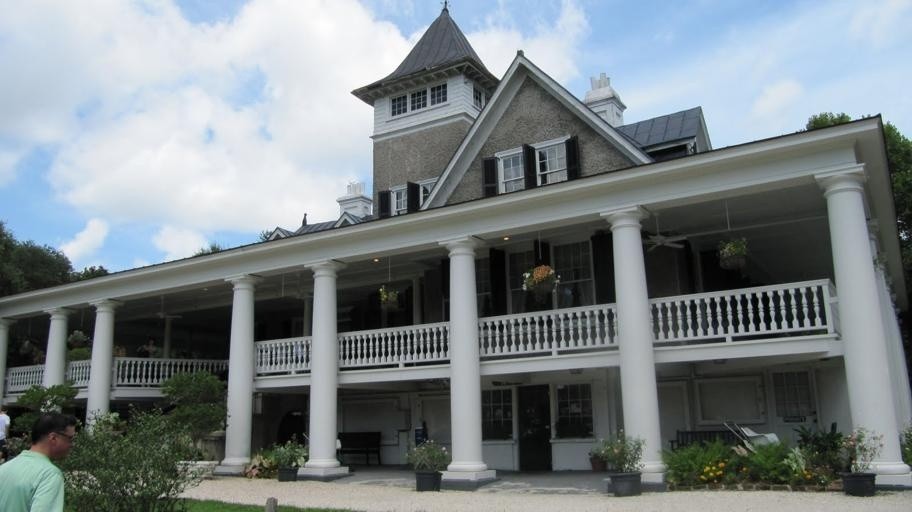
[
  {"xmin": 137, "ymin": 335, "xmax": 157, "ymax": 358},
  {"xmin": 0, "ymin": 408, "xmax": 11, "ymax": 462},
  {"xmin": 0, "ymin": 409, "xmax": 77, "ymax": 512}
]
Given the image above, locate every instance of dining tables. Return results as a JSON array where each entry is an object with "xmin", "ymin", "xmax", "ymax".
[
  {"xmin": 713, "ymin": 201, "xmax": 751, "ymax": 273},
  {"xmin": 158, "ymin": 369, "xmax": 232, "ymax": 480},
  {"xmin": 271, "ymin": 438, "xmax": 309, "ymax": 482},
  {"xmin": 377, "ymin": 255, "xmax": 402, "ymax": 313}
]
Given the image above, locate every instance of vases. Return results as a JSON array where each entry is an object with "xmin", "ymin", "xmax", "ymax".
[
  {"xmin": 834, "ymin": 422, "xmax": 885, "ymax": 474},
  {"xmin": 522, "ymin": 230, "xmax": 563, "ymax": 297},
  {"xmin": 404, "ymin": 437, "xmax": 453, "ymax": 473},
  {"xmin": 588, "ymin": 425, "xmax": 648, "ymax": 472},
  {"xmin": 700, "ymin": 456, "xmax": 727, "ymax": 483}
]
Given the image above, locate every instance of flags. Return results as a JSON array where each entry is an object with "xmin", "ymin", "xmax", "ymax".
[
  {"xmin": 840, "ymin": 476, "xmax": 879, "ymax": 498},
  {"xmin": 415, "ymin": 474, "xmax": 443, "ymax": 491},
  {"xmin": 610, "ymin": 474, "xmax": 643, "ymax": 495}
]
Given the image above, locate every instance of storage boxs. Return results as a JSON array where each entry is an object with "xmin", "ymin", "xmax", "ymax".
[
  {"xmin": 670, "ymin": 428, "xmax": 746, "ymax": 452},
  {"xmin": 337, "ymin": 429, "xmax": 383, "ymax": 467}
]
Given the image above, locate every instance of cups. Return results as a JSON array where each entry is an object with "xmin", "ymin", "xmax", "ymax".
[{"xmin": 55, "ymin": 430, "xmax": 76, "ymax": 443}]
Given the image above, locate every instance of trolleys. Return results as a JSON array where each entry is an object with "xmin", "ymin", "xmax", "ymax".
[{"xmin": 642, "ymin": 208, "xmax": 689, "ymax": 255}]
